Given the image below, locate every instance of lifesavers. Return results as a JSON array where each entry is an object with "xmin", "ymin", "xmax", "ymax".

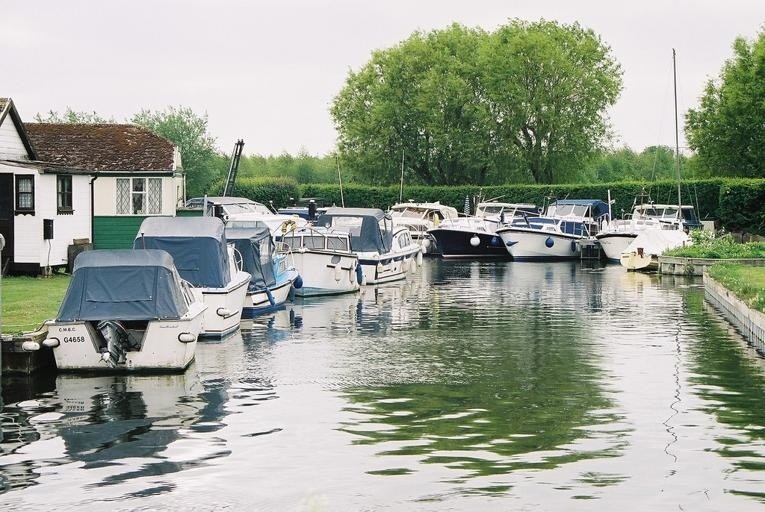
[{"xmin": 281, "ymin": 219, "xmax": 296, "ymax": 232}]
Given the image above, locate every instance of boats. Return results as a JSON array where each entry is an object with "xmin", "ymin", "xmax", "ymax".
[
  {"xmin": 619, "ymin": 229, "xmax": 695, "ymax": 272},
  {"xmin": 377, "ymin": 199, "xmax": 609, "ymax": 262}
]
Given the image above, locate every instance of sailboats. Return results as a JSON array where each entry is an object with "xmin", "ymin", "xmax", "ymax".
[{"xmin": 593, "ymin": 50, "xmax": 703, "ymax": 261}]
